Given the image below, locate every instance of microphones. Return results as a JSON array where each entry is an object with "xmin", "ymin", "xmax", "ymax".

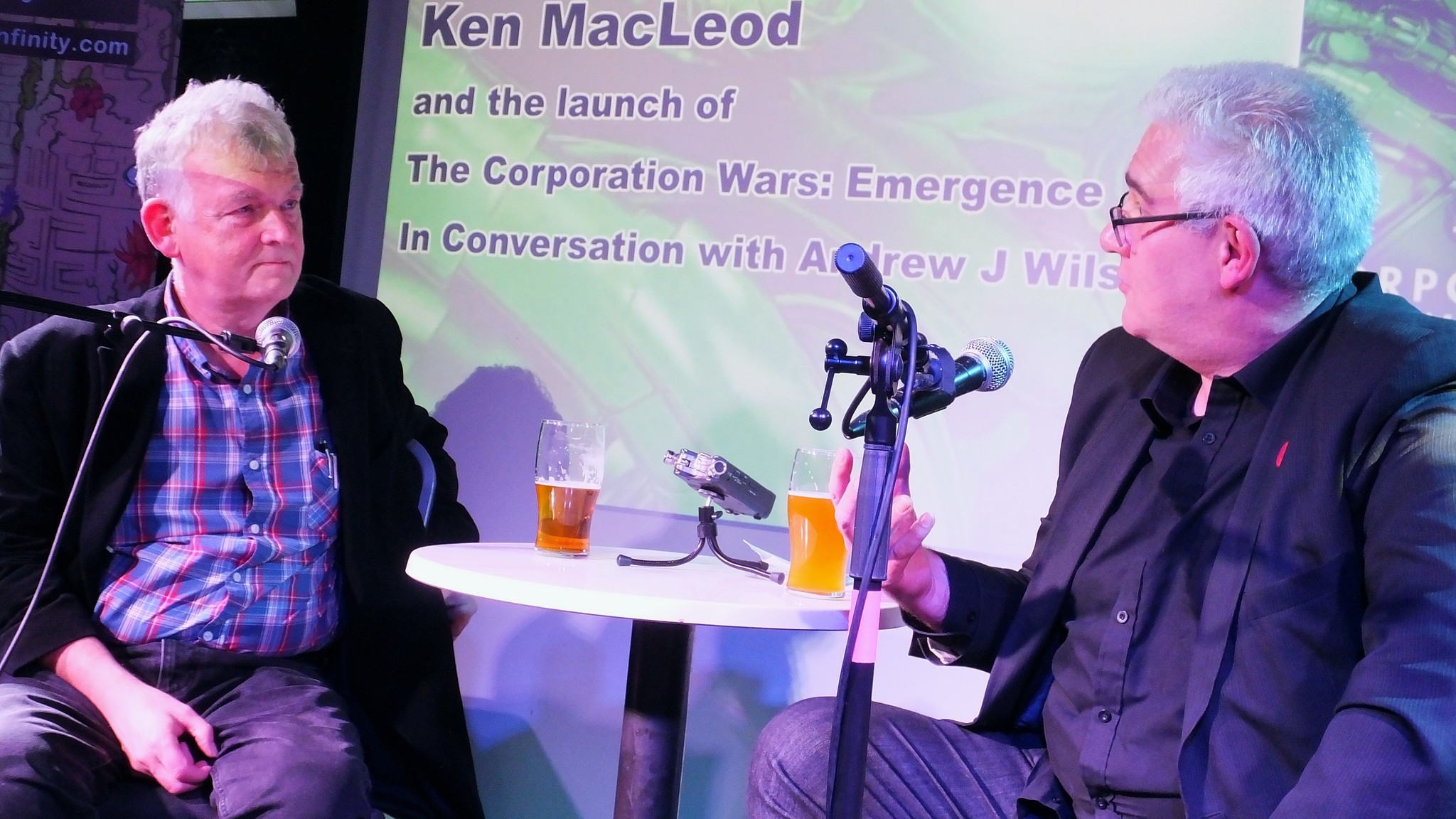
[
  {"xmin": 255, "ymin": 317, "xmax": 302, "ymax": 373},
  {"xmin": 842, "ymin": 338, "xmax": 1015, "ymax": 440}
]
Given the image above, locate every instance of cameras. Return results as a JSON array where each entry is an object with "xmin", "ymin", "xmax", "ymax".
[{"xmin": 675, "ymin": 448, "xmax": 776, "ymax": 520}]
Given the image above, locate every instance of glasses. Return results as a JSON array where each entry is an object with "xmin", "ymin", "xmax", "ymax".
[{"xmin": 1109, "ymin": 191, "xmax": 1230, "ymax": 248}]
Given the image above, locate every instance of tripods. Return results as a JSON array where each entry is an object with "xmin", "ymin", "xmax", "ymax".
[{"xmin": 615, "ymin": 505, "xmax": 785, "ymax": 585}]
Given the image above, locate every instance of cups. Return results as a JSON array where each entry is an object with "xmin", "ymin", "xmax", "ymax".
[
  {"xmin": 533, "ymin": 419, "xmax": 606, "ymax": 559},
  {"xmin": 785, "ymin": 448, "xmax": 862, "ymax": 600}
]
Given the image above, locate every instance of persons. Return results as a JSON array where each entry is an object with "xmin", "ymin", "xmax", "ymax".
[
  {"xmin": 0, "ymin": 79, "xmax": 481, "ymax": 819},
  {"xmin": 747, "ymin": 63, "xmax": 1456, "ymax": 819}
]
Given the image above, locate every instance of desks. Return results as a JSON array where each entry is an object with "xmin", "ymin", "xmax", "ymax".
[{"xmin": 403, "ymin": 540, "xmax": 908, "ymax": 819}]
